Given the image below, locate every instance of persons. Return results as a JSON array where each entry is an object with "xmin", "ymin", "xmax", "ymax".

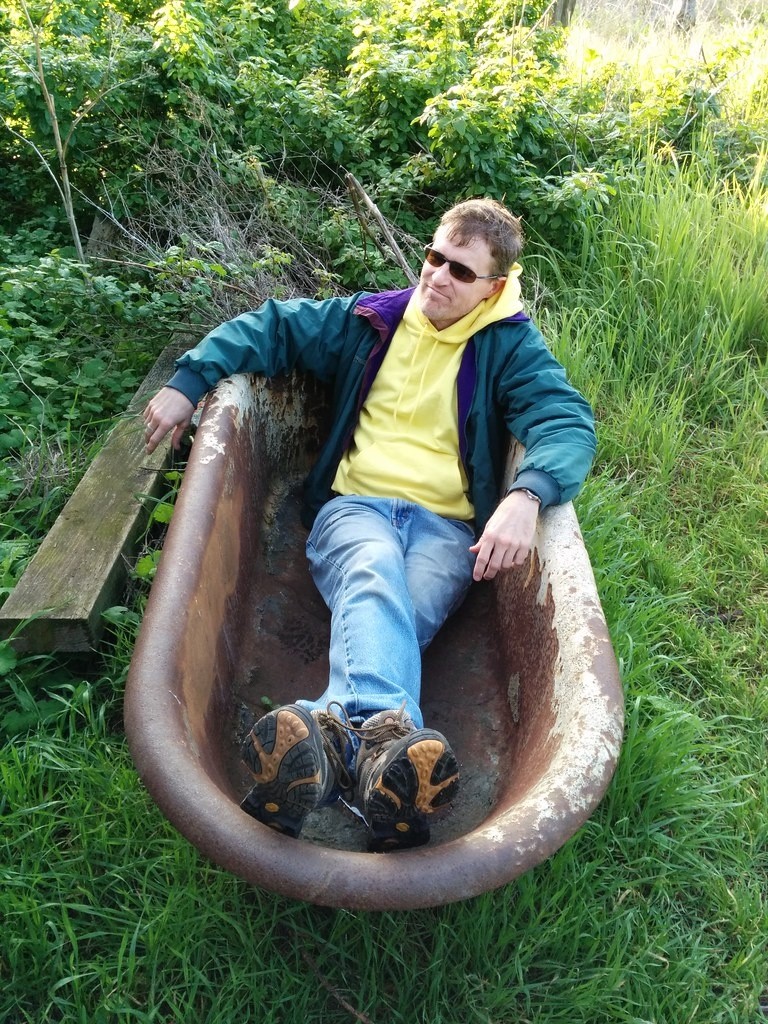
[{"xmin": 143, "ymin": 196, "xmax": 598, "ymax": 850}]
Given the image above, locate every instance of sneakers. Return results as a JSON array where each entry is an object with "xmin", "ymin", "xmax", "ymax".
[
  {"xmin": 326, "ymin": 696, "xmax": 458, "ymax": 853},
  {"xmin": 239, "ymin": 705, "xmax": 353, "ymax": 838}
]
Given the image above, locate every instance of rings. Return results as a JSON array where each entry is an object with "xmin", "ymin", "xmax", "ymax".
[{"xmin": 146, "ymin": 423, "xmax": 154, "ymax": 432}]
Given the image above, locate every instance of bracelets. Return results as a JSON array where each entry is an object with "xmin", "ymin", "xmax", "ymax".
[{"xmin": 520, "ymin": 488, "xmax": 542, "ymax": 503}]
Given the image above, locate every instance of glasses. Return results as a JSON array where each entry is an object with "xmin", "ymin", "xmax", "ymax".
[{"xmin": 421, "ymin": 241, "xmax": 499, "ymax": 284}]
